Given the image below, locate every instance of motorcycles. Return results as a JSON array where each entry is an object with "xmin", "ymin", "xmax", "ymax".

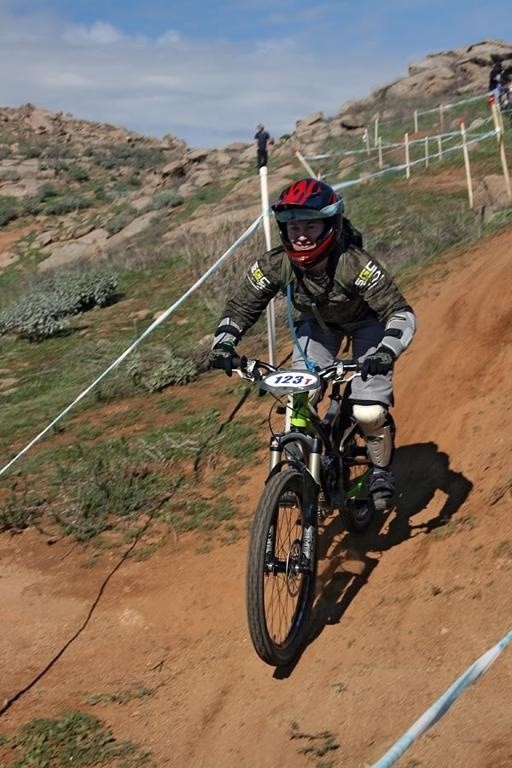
[{"xmin": 196, "ymin": 352, "xmax": 389, "ymax": 667}]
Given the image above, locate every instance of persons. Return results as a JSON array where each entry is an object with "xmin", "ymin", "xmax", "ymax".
[
  {"xmin": 253, "ymin": 123, "xmax": 270, "ymax": 170},
  {"xmin": 207, "ymin": 177, "xmax": 417, "ymax": 511},
  {"xmin": 486, "ymin": 60, "xmax": 512, "ymax": 128}
]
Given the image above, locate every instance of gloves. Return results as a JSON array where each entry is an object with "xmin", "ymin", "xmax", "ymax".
[
  {"xmin": 361, "ymin": 344, "xmax": 395, "ymax": 383},
  {"xmin": 208, "ymin": 343, "xmax": 241, "ymax": 377}
]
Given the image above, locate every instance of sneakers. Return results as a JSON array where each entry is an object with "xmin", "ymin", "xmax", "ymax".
[
  {"xmin": 277, "ymin": 490, "xmax": 298, "ymax": 508},
  {"xmin": 365, "ymin": 467, "xmax": 396, "ymax": 511}
]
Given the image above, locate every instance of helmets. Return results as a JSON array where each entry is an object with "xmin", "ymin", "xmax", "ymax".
[{"xmin": 270, "ymin": 178, "xmax": 344, "ymax": 271}]
